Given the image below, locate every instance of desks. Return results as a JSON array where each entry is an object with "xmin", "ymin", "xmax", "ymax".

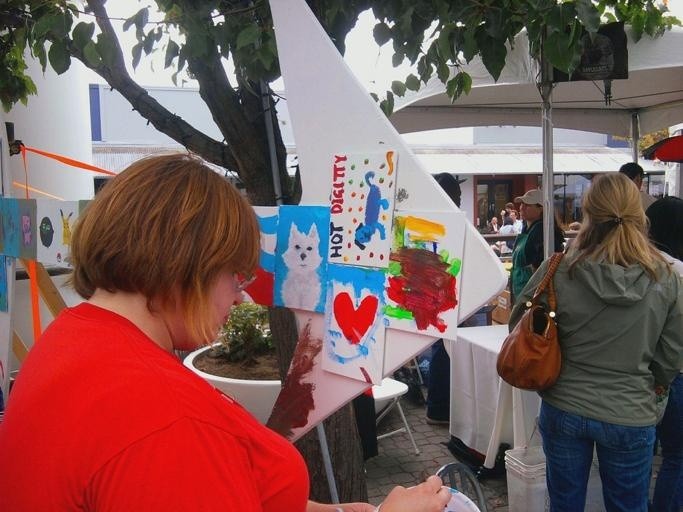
[{"xmin": 445, "ymin": 322, "xmax": 543, "ymax": 481}]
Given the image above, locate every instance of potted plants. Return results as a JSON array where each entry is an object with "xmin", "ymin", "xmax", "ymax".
[{"xmin": 180, "ymin": 304, "xmax": 282, "ymax": 426}]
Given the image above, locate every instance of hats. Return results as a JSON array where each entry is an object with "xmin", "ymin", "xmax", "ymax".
[{"xmin": 514, "ymin": 189, "xmax": 544, "ymax": 205}]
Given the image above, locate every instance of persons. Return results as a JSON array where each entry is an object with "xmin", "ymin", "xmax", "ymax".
[
  {"xmin": 511, "ymin": 189, "xmax": 566, "ymax": 312},
  {"xmin": 570, "ymin": 221, "xmax": 581, "ymax": 231},
  {"xmin": 645, "ymin": 196, "xmax": 683, "ymax": 278},
  {"xmin": 618, "ymin": 163, "xmax": 658, "ymax": 212},
  {"xmin": 1, "ymin": 150, "xmax": 454, "ymax": 511},
  {"xmin": 480, "ymin": 202, "xmax": 523, "ymax": 256},
  {"xmin": 426, "ymin": 172, "xmax": 462, "ymax": 426},
  {"xmin": 514, "ymin": 173, "xmax": 683, "ymax": 512}
]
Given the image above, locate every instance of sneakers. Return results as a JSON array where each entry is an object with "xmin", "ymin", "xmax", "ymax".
[{"xmin": 424, "ymin": 415, "xmax": 450, "ymax": 424}]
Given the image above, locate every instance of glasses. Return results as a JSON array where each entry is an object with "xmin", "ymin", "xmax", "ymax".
[{"xmin": 232, "ymin": 265, "xmax": 257, "ymax": 291}]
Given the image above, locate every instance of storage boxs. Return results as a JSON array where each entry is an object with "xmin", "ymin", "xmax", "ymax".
[{"xmin": 491, "ymin": 290, "xmax": 513, "ymax": 325}]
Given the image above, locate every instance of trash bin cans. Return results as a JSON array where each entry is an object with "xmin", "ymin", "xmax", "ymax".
[{"xmin": 504, "ymin": 445, "xmax": 607, "ymax": 512}]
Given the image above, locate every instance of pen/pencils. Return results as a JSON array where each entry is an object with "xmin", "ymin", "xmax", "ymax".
[{"xmin": 420, "ymin": 469, "xmax": 427, "ymax": 483}]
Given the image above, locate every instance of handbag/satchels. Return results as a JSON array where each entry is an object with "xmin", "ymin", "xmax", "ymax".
[{"xmin": 497, "ymin": 304, "xmax": 561, "ymax": 391}]
